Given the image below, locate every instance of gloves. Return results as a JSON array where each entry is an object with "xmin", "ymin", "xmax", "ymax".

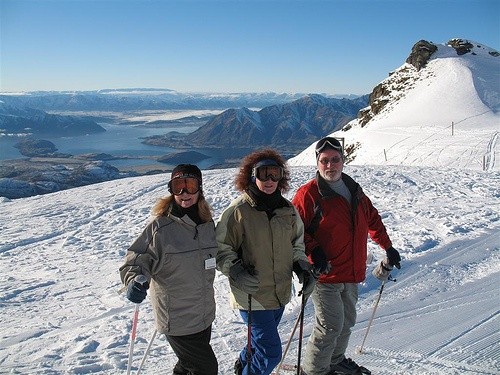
[
  {"xmin": 126, "ymin": 279, "xmax": 150, "ymax": 304},
  {"xmin": 312, "ymin": 247, "xmax": 332, "ymax": 274},
  {"xmin": 228, "ymin": 262, "xmax": 260, "ymax": 295},
  {"xmin": 299, "ymin": 270, "xmax": 316, "ymax": 306},
  {"xmin": 386, "ymin": 248, "xmax": 402, "ymax": 270}
]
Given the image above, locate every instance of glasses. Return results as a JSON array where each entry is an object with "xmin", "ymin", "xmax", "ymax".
[
  {"xmin": 319, "ymin": 156, "xmax": 342, "ymax": 165},
  {"xmin": 315, "ymin": 137, "xmax": 343, "ymax": 158},
  {"xmin": 251, "ymin": 163, "xmax": 284, "ymax": 182},
  {"xmin": 168, "ymin": 174, "xmax": 201, "ymax": 196}
]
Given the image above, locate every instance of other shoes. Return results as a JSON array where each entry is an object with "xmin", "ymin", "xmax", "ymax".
[{"xmin": 332, "ymin": 358, "xmax": 371, "ymax": 375}]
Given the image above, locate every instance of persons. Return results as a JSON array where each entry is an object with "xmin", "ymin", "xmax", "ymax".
[
  {"xmin": 215, "ymin": 149, "xmax": 316, "ymax": 375},
  {"xmin": 291, "ymin": 137, "xmax": 401, "ymax": 375},
  {"xmin": 119, "ymin": 163, "xmax": 218, "ymax": 375}
]
